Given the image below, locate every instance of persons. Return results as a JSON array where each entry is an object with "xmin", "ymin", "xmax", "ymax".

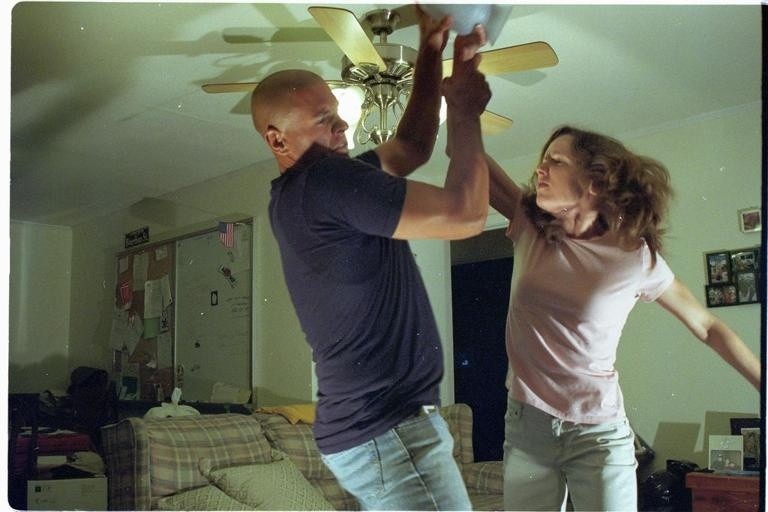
[
  {"xmin": 713, "ymin": 261, "xmax": 727, "ymax": 283},
  {"xmin": 711, "ymin": 452, "xmax": 726, "ymax": 469},
  {"xmin": 253, "ymin": 5, "xmax": 493, "ymax": 511},
  {"xmin": 443, "ymin": 21, "xmax": 763, "ymax": 511},
  {"xmin": 724, "ymin": 457, "xmax": 737, "ymax": 467}
]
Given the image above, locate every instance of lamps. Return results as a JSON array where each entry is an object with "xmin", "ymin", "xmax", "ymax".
[{"xmin": 331, "ymin": 75, "xmax": 448, "ymax": 149}]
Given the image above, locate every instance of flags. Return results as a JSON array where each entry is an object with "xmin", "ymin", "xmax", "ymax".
[{"xmin": 218, "ymin": 221, "xmax": 234, "ymax": 248}]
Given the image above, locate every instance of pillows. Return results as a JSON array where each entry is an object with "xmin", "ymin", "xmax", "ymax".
[{"xmin": 159, "ymin": 453, "xmax": 337, "ymax": 512}]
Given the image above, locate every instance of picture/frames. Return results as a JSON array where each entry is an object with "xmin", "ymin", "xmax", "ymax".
[
  {"xmin": 702, "ymin": 247, "xmax": 764, "ymax": 308},
  {"xmin": 706, "ymin": 415, "xmax": 762, "ymax": 477}
]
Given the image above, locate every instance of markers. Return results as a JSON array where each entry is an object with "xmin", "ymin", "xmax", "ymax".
[{"xmin": 180, "ymin": 399, "xmax": 200, "ymax": 403}]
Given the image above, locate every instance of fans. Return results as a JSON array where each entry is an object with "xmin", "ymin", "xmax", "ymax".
[{"xmin": 203, "ymin": 5, "xmax": 561, "ymax": 137}]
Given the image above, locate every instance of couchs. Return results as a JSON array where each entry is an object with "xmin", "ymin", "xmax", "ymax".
[{"xmin": 96, "ymin": 403, "xmax": 505, "ymax": 511}]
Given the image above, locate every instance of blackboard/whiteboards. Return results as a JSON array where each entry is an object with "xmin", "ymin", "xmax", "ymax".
[{"xmin": 112, "ymin": 214, "xmax": 259, "ymax": 406}]
